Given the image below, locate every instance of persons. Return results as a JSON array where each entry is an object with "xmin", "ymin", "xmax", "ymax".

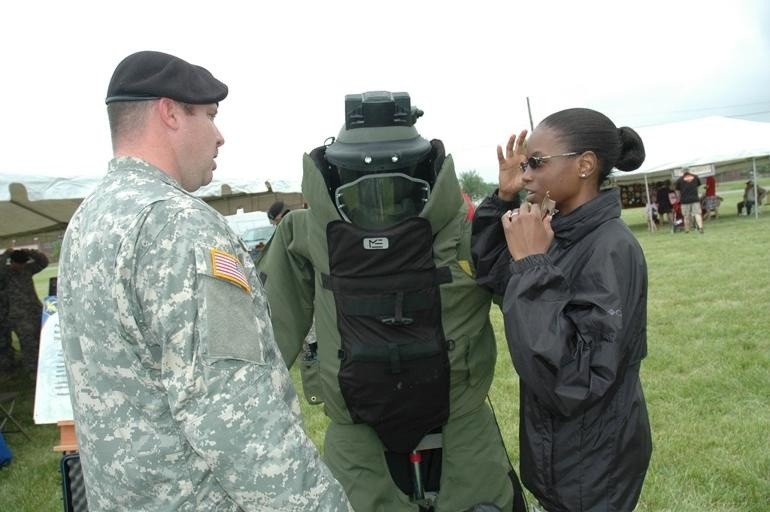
[
  {"xmin": 737, "ymin": 180, "xmax": 768, "ymax": 216},
  {"xmin": 268, "ymin": 198, "xmax": 318, "ymax": 369},
  {"xmin": 0, "ymin": 246, "xmax": 52, "ymax": 388},
  {"xmin": 1, "ymin": 313, "xmax": 18, "ymax": 357},
  {"xmin": 55, "ymin": 48, "xmax": 355, "ymax": 512},
  {"xmin": 646, "ymin": 164, "xmax": 723, "ymax": 234},
  {"xmin": 469, "ymin": 105, "xmax": 656, "ymax": 511}
]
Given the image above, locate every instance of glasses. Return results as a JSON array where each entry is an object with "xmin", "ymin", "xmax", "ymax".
[{"xmin": 517, "ymin": 150, "xmax": 584, "ymax": 173}]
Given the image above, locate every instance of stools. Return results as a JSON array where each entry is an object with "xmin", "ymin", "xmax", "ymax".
[{"xmin": 0, "ymin": 391, "xmax": 36, "ymax": 443}]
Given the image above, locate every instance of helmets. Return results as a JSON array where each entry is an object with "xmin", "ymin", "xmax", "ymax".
[{"xmin": 325, "ymin": 90, "xmax": 432, "ymax": 209}]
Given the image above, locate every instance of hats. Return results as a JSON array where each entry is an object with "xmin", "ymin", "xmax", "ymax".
[{"xmin": 102, "ymin": 50, "xmax": 230, "ymax": 106}]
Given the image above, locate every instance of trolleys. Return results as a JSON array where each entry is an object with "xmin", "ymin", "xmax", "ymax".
[{"xmin": 672, "ymin": 199, "xmax": 699, "ymax": 234}]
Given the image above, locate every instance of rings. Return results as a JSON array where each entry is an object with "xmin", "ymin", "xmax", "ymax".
[{"xmin": 511, "ymin": 212, "xmax": 520, "ymax": 217}]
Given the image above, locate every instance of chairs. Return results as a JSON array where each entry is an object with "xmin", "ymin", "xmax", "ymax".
[
  {"xmin": 60, "ymin": 454, "xmax": 88, "ymax": 512},
  {"xmin": 744, "ymin": 190, "xmax": 766, "ymax": 216}
]
